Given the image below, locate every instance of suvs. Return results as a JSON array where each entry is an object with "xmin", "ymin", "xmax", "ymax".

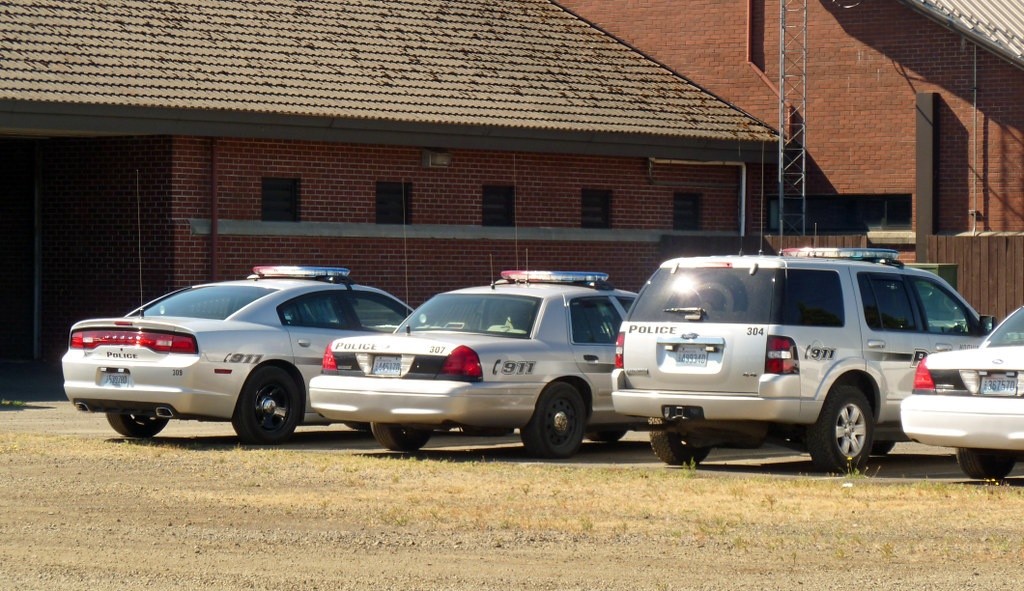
[{"xmin": 610, "ymin": 249, "xmax": 1000, "ymax": 472}]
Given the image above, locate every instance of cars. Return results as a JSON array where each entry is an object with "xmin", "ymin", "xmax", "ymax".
[
  {"xmin": 308, "ymin": 267, "xmax": 643, "ymax": 460},
  {"xmin": 901, "ymin": 307, "xmax": 1024, "ymax": 475},
  {"xmin": 61, "ymin": 265, "xmax": 421, "ymax": 446}
]
